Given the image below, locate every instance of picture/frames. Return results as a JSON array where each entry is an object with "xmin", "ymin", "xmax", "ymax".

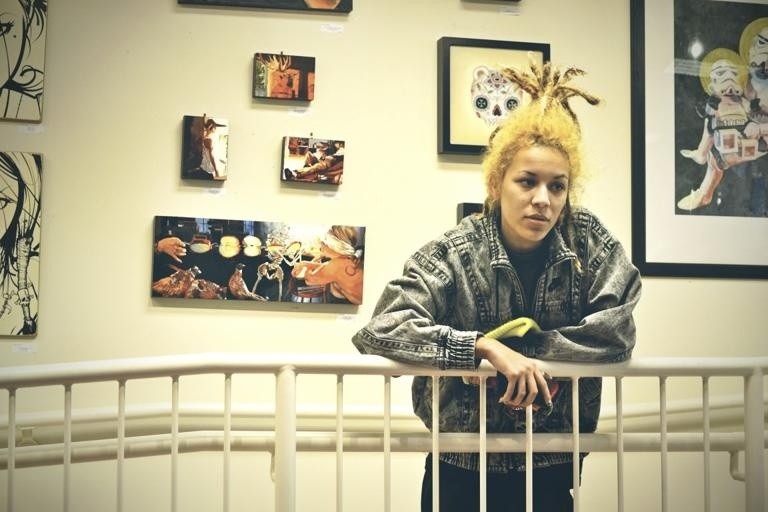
[
  {"xmin": 437, "ymin": 37, "xmax": 549, "ymax": 153},
  {"xmin": 631, "ymin": 0, "xmax": 768, "ymax": 279}
]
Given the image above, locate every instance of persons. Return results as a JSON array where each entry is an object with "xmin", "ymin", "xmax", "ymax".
[
  {"xmin": 200, "ymin": 119, "xmax": 219, "ymax": 179},
  {"xmin": 0, "ymin": 0, "xmax": 46, "ymax": 120},
  {"xmin": 350, "ymin": 57, "xmax": 642, "ymax": 512},
  {"xmin": 0, "ymin": 151, "xmax": 43, "ymax": 338},
  {"xmin": 291, "ymin": 225, "xmax": 364, "ymax": 305},
  {"xmin": 285, "ymin": 141, "xmax": 344, "ymax": 181},
  {"xmin": 677, "ymin": 17, "xmax": 768, "ymax": 211},
  {"xmin": 154, "ymin": 236, "xmax": 187, "ymax": 263}
]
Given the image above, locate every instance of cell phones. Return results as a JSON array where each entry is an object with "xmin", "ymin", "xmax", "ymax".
[{"xmin": 506, "ymin": 381, "xmax": 560, "ymax": 408}]
